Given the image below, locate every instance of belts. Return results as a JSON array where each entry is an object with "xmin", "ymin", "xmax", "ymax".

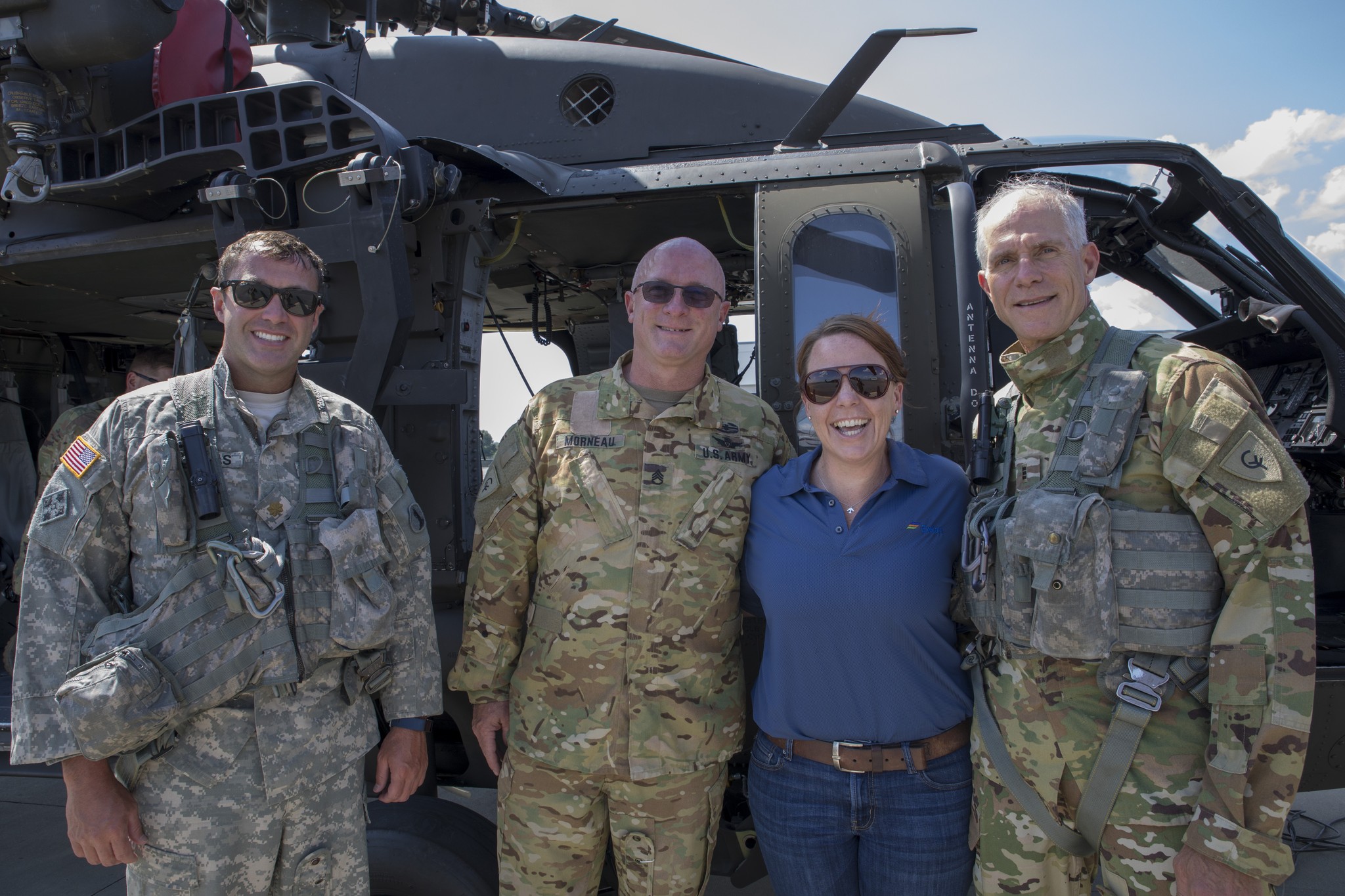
[{"xmin": 764, "ymin": 721, "xmax": 971, "ymax": 774}]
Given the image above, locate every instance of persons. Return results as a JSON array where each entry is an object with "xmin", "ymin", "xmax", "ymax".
[
  {"xmin": 10, "ymin": 234, "xmax": 443, "ymax": 896},
  {"xmin": 449, "ymin": 240, "xmax": 800, "ymax": 896},
  {"xmin": 740, "ymin": 316, "xmax": 977, "ymax": 896},
  {"xmin": 5, "ymin": 347, "xmax": 186, "ymax": 680},
  {"xmin": 949, "ymin": 182, "xmax": 1317, "ymax": 896}
]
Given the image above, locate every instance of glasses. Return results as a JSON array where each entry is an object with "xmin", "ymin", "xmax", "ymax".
[
  {"xmin": 798, "ymin": 363, "xmax": 895, "ymax": 404},
  {"xmin": 631, "ymin": 280, "xmax": 723, "ymax": 309},
  {"xmin": 220, "ymin": 280, "xmax": 322, "ymax": 319}
]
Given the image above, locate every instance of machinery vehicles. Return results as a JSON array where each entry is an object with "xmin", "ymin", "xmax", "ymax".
[{"xmin": 1, "ymin": 0, "xmax": 1345, "ymax": 895}]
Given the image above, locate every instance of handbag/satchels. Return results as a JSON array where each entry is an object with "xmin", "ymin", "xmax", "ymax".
[{"xmin": 52, "ymin": 644, "xmax": 186, "ymax": 761}]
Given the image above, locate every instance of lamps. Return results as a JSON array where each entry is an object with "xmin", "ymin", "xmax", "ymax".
[{"xmin": 576, "ymin": 270, "xmax": 592, "ymax": 288}]
